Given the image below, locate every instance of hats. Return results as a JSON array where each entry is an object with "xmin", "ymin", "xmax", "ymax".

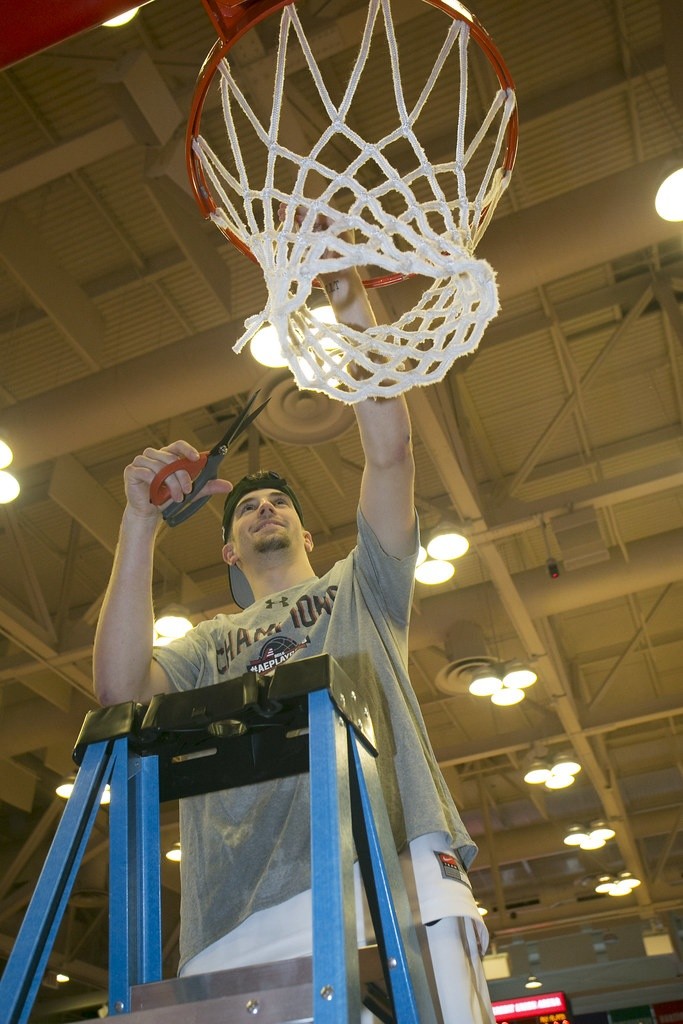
[{"xmin": 221, "ymin": 471, "xmax": 304, "ymax": 610}]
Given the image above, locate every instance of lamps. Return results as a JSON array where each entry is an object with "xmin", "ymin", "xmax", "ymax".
[
  {"xmin": 249, "ymin": 296, "xmax": 344, "ymax": 388},
  {"xmin": 166, "ymin": 840, "xmax": 181, "ymax": 862},
  {"xmin": 525, "ymin": 973, "xmax": 543, "ymax": 989},
  {"xmin": 564, "ymin": 819, "xmax": 616, "ymax": 850},
  {"xmin": 155, "ymin": 604, "xmax": 193, "ymax": 637},
  {"xmin": 523, "ymin": 752, "xmax": 581, "ymax": 790},
  {"xmin": 56, "ymin": 773, "xmax": 77, "ymax": 799},
  {"xmin": 415, "ymin": 521, "xmax": 470, "ymax": 586},
  {"xmin": 595, "ymin": 870, "xmax": 640, "ymax": 897},
  {"xmin": 468, "ymin": 559, "xmax": 538, "ymax": 706},
  {"xmin": 604, "ymin": 15, "xmax": 683, "ymax": 223}
]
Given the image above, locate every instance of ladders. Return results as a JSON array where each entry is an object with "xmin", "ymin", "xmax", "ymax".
[{"xmin": 0, "ymin": 647, "xmax": 448, "ymax": 1023}]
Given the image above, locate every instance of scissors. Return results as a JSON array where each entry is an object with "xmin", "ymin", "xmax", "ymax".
[{"xmin": 149, "ymin": 388, "xmax": 273, "ymax": 528}]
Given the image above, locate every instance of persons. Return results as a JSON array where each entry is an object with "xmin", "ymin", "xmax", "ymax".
[{"xmin": 91, "ymin": 201, "xmax": 494, "ymax": 1024}]
